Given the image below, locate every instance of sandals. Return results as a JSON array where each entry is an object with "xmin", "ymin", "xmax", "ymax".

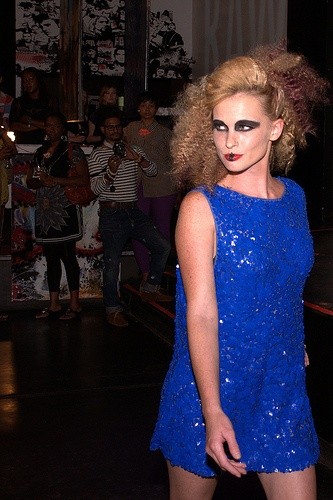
[
  {"xmin": 34, "ymin": 306, "xmax": 63, "ymax": 319},
  {"xmin": 60, "ymin": 304, "xmax": 83, "ymax": 319}
]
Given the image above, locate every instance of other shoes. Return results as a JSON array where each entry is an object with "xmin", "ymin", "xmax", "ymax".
[
  {"xmin": 108, "ymin": 313, "xmax": 129, "ymax": 327},
  {"xmin": 138, "ymin": 282, "xmax": 175, "ymax": 303}
]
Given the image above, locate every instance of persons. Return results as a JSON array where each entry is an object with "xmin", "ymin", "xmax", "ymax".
[
  {"xmin": 149, "ymin": 38, "xmax": 320, "ymax": 500},
  {"xmin": 0, "ymin": 66, "xmax": 177, "ymax": 327}
]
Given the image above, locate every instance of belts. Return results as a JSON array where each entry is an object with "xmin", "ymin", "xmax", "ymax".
[{"xmin": 101, "ymin": 201, "xmax": 137, "ymax": 209}]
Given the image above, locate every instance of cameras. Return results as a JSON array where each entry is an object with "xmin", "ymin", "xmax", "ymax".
[{"xmin": 113, "ymin": 142, "xmax": 127, "ymax": 159}]
